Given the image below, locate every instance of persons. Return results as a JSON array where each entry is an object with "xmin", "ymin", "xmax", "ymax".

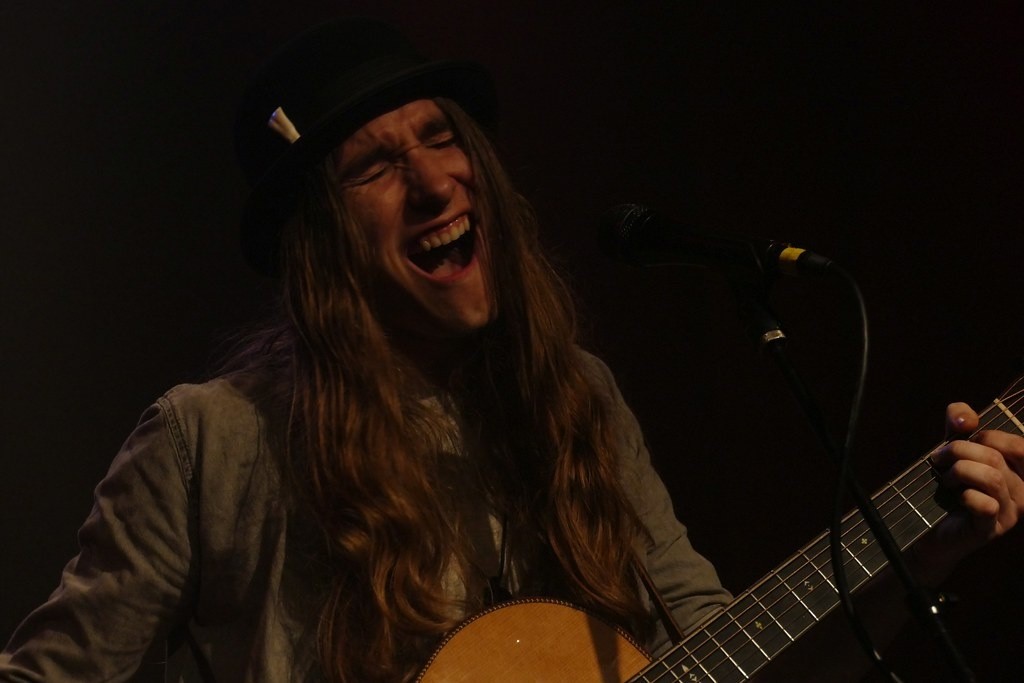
[{"xmin": 0, "ymin": 20, "xmax": 1024, "ymax": 683}]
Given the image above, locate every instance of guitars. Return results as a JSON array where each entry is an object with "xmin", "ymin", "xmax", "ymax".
[{"xmin": 415, "ymin": 381, "xmax": 1024, "ymax": 681}]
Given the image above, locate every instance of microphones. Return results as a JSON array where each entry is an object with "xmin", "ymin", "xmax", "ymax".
[{"xmin": 596, "ymin": 204, "xmax": 836, "ymax": 277}]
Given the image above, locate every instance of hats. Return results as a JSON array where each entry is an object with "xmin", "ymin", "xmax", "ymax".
[{"xmin": 236, "ymin": 16, "xmax": 501, "ymax": 271}]
